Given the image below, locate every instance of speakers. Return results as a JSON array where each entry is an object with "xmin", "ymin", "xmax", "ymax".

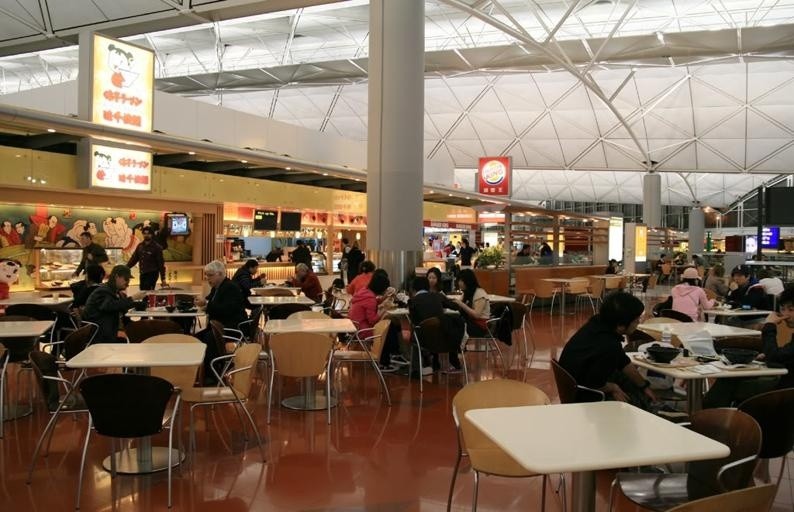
[{"xmin": 766, "ymin": 187, "xmax": 794, "ymax": 226}]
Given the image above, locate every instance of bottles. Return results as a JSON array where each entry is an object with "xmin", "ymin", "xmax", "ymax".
[{"xmin": 660, "ymin": 325, "xmax": 672, "ymax": 345}]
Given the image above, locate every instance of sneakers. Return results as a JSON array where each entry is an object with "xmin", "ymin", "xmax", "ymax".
[
  {"xmin": 378, "ymin": 365, "xmax": 399, "ymax": 373},
  {"xmin": 389, "ymin": 353, "xmax": 410, "ymax": 365},
  {"xmin": 422, "ymin": 367, "xmax": 435, "ymax": 375},
  {"xmin": 439, "ymin": 363, "xmax": 463, "ymax": 374}
]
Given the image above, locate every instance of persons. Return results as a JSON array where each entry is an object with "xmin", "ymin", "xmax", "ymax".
[
  {"xmin": 512, "ymin": 244, "xmax": 533, "ymax": 267},
  {"xmin": 0, "ymin": 217, "xmax": 141, "ymax": 258},
  {"xmin": 193, "ymin": 261, "xmax": 248, "ymax": 386},
  {"xmin": 606, "ymin": 252, "xmax": 794, "ymax": 395},
  {"xmin": 702, "ymin": 287, "xmax": 794, "ymax": 409},
  {"xmin": 232, "ymin": 259, "xmax": 266, "ymax": 343},
  {"xmin": 69, "ymin": 264, "xmax": 106, "ymax": 311},
  {"xmin": 558, "ymin": 292, "xmax": 658, "ymax": 406},
  {"xmin": 71, "ymin": 231, "xmax": 109, "ymax": 280},
  {"xmin": 81, "ymin": 264, "xmax": 136, "ymax": 344},
  {"xmin": 538, "ymin": 241, "xmax": 551, "ymax": 257},
  {"xmin": 126, "ymin": 225, "xmax": 167, "ymax": 292},
  {"xmin": 267, "ymin": 225, "xmax": 490, "ymax": 381}
]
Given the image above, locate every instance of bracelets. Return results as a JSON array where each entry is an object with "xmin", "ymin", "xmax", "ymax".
[
  {"xmin": 640, "ymin": 379, "xmax": 649, "ymax": 391},
  {"xmin": 205, "ymin": 300, "xmax": 209, "ymax": 305}
]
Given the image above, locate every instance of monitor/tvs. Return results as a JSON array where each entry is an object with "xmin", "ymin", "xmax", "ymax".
[
  {"xmin": 167, "ymin": 214, "xmax": 189, "ymax": 236},
  {"xmin": 281, "ymin": 211, "xmax": 301, "ymax": 231},
  {"xmin": 254, "ymin": 210, "xmax": 277, "ymax": 230}
]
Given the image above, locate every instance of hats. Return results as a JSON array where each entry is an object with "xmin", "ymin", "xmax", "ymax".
[
  {"xmin": 111, "ymin": 265, "xmax": 135, "ymax": 279},
  {"xmin": 683, "ymin": 267, "xmax": 703, "ymax": 281},
  {"xmin": 732, "ymin": 264, "xmax": 750, "ymax": 274}
]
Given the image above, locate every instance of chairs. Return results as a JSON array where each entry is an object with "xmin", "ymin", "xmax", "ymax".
[
  {"xmin": 540, "ymin": 259, "xmax": 794, "ymax": 402},
  {"xmin": 447, "ymin": 378, "xmax": 567, "ymax": 510},
  {"xmin": 608, "ymin": 387, "xmax": 794, "ymax": 511}
]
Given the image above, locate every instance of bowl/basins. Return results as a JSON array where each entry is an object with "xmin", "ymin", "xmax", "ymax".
[
  {"xmin": 721, "ymin": 347, "xmax": 759, "ymax": 367},
  {"xmin": 134, "ymin": 300, "xmax": 195, "ymax": 312},
  {"xmin": 646, "ymin": 346, "xmax": 681, "ymax": 363}
]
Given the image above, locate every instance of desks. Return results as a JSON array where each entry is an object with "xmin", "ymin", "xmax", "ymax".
[{"xmin": 463, "ymin": 399, "xmax": 732, "ymax": 512}]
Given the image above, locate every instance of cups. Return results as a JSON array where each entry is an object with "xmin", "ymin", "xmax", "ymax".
[{"xmin": 296, "ymin": 287, "xmax": 301, "ymax": 295}]
